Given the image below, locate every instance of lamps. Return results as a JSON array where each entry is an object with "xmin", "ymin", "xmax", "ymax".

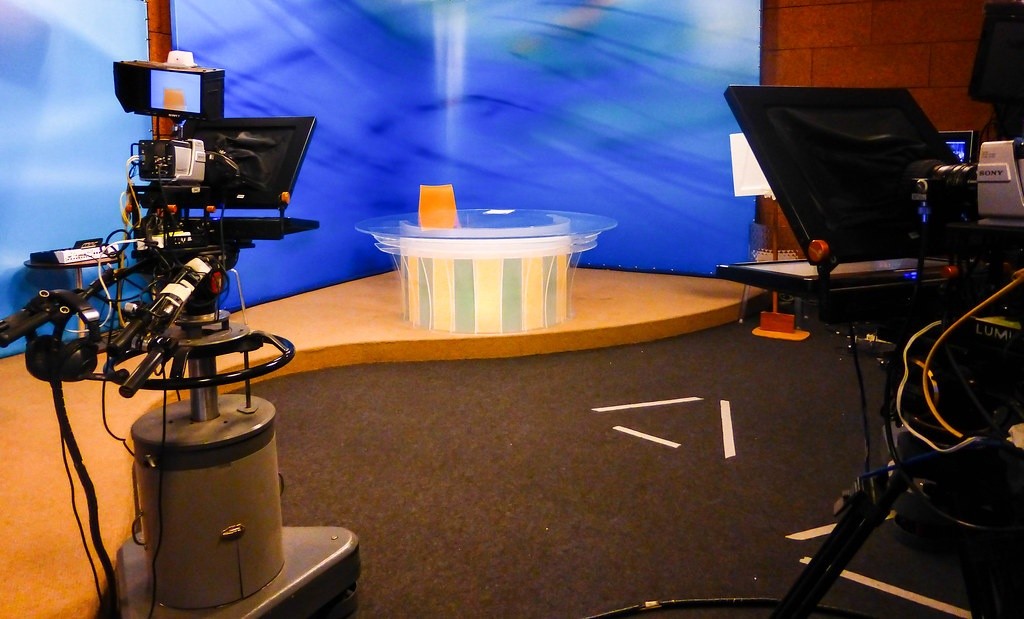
[{"xmin": 728, "ymin": 131, "xmax": 812, "ymax": 342}]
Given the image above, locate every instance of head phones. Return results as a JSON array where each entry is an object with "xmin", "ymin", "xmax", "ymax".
[{"xmin": 22, "ymin": 288, "xmax": 101, "ymax": 383}]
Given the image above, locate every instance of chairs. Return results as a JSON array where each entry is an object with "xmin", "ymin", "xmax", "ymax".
[{"xmin": 418, "ymin": 184, "xmax": 462, "ymax": 230}]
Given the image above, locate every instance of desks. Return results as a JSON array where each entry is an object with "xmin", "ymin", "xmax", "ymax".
[{"xmin": 354, "ymin": 209, "xmax": 618, "ymax": 336}]
